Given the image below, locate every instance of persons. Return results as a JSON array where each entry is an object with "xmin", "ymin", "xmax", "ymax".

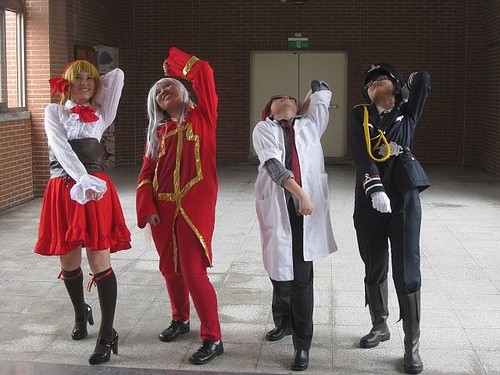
[
  {"xmin": 135, "ymin": 46, "xmax": 224, "ymax": 365},
  {"xmin": 252, "ymin": 80, "xmax": 338, "ymax": 371},
  {"xmin": 347, "ymin": 64, "xmax": 430, "ymax": 375},
  {"xmin": 34, "ymin": 61, "xmax": 131, "ymax": 365}
]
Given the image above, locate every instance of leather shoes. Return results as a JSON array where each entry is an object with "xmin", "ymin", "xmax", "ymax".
[
  {"xmin": 291, "ymin": 348, "xmax": 309, "ymax": 372},
  {"xmin": 158, "ymin": 318, "xmax": 191, "ymax": 342},
  {"xmin": 188, "ymin": 340, "xmax": 224, "ymax": 365},
  {"xmin": 266, "ymin": 326, "xmax": 293, "ymax": 341}
]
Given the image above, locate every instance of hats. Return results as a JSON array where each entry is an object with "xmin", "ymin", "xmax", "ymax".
[{"xmin": 362, "ymin": 63, "xmax": 403, "ymax": 88}]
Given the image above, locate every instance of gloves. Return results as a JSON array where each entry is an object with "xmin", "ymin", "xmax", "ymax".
[
  {"xmin": 372, "ymin": 192, "xmax": 392, "ymax": 214},
  {"xmin": 401, "ymin": 84, "xmax": 409, "ymax": 99}
]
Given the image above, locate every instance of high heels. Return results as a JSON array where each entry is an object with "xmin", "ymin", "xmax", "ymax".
[
  {"xmin": 70, "ymin": 304, "xmax": 94, "ymax": 340},
  {"xmin": 88, "ymin": 328, "xmax": 118, "ymax": 365}
]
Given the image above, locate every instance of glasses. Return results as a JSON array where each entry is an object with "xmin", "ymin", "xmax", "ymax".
[{"xmin": 363, "ymin": 75, "xmax": 391, "ymax": 90}]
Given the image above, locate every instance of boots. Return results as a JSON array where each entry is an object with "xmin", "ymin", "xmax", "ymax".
[
  {"xmin": 398, "ymin": 291, "xmax": 424, "ymax": 375},
  {"xmin": 359, "ymin": 276, "xmax": 391, "ymax": 349}
]
